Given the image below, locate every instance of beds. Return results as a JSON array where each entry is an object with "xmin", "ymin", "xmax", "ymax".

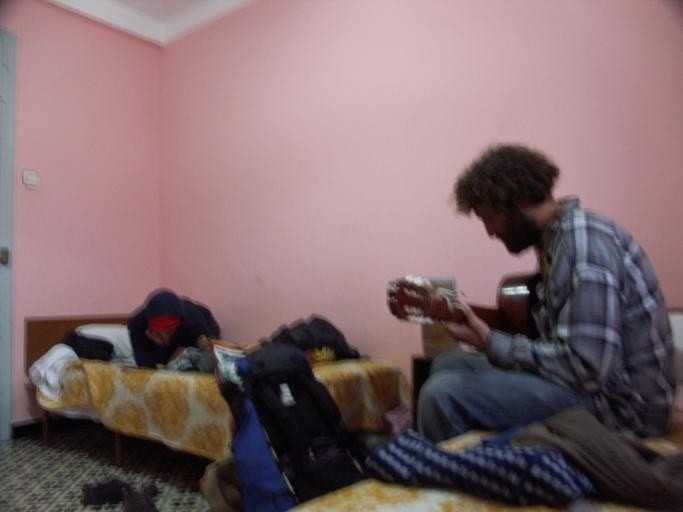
[
  {"xmin": 24, "ymin": 315, "xmax": 410, "ymax": 467},
  {"xmin": 284, "ymin": 275, "xmax": 683, "ymax": 511}
]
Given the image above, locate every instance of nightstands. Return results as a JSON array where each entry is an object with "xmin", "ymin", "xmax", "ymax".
[{"xmin": 410, "ymin": 354, "xmax": 434, "ymax": 432}]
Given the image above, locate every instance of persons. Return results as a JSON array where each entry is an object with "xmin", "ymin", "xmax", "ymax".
[
  {"xmin": 128, "ymin": 289, "xmax": 221, "ymax": 369},
  {"xmin": 414, "ymin": 140, "xmax": 675, "ymax": 445}
]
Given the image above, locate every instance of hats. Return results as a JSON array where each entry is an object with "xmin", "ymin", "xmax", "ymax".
[{"xmin": 140, "ymin": 289, "xmax": 184, "ymax": 334}]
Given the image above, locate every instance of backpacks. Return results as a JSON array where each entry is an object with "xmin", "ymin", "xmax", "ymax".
[{"xmin": 212, "ymin": 341, "xmax": 373, "ymax": 512}]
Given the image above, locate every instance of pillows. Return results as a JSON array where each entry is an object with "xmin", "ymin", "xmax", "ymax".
[{"xmin": 77, "ymin": 322, "xmax": 134, "ymax": 366}]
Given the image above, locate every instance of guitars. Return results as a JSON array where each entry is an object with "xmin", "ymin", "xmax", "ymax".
[{"xmin": 384, "ymin": 273, "xmax": 542, "ymax": 338}]
